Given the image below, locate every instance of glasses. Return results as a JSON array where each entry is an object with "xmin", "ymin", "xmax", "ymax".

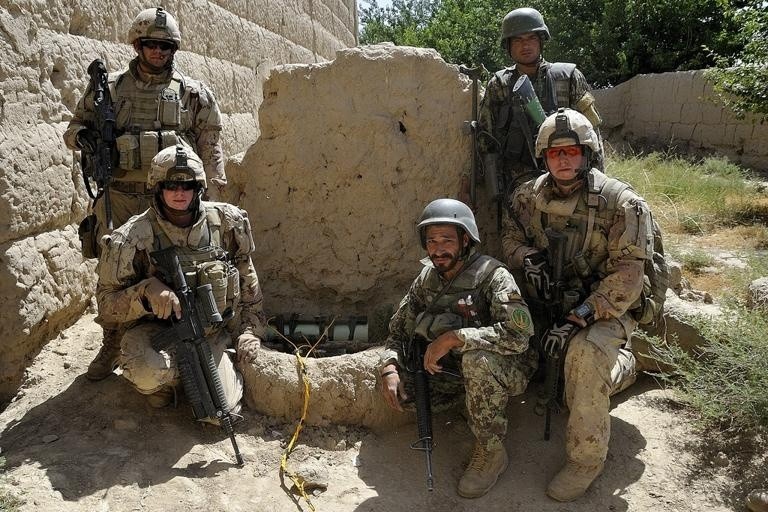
[
  {"xmin": 543, "ymin": 147, "xmax": 582, "ymax": 159},
  {"xmin": 142, "ymin": 40, "xmax": 173, "ymax": 52},
  {"xmin": 158, "ymin": 180, "xmax": 197, "ymax": 191}
]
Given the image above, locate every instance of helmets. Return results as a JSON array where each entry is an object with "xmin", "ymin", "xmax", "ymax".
[
  {"xmin": 417, "ymin": 198, "xmax": 482, "ymax": 248},
  {"xmin": 147, "ymin": 143, "xmax": 208, "ymax": 191},
  {"xmin": 533, "ymin": 107, "xmax": 601, "ymax": 157},
  {"xmin": 500, "ymin": 7, "xmax": 551, "ymax": 45},
  {"xmin": 127, "ymin": 7, "xmax": 182, "ymax": 44}
]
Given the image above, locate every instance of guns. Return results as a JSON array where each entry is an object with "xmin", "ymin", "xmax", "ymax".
[
  {"xmin": 531, "ymin": 229, "xmax": 580, "ymax": 442},
  {"xmin": 400, "ymin": 332, "xmax": 463, "ymax": 492},
  {"xmin": 81, "ymin": 59, "xmax": 117, "ymax": 231},
  {"xmin": 141, "ymin": 246, "xmax": 244, "ymax": 468},
  {"xmin": 512, "ymin": 74, "xmax": 547, "ymax": 170}
]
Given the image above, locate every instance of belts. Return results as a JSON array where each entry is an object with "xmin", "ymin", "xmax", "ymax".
[{"xmin": 110, "ymin": 180, "xmax": 152, "ymax": 196}]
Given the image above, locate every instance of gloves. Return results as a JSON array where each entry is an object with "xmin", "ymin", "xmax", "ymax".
[
  {"xmin": 525, "ymin": 253, "xmax": 553, "ymax": 299},
  {"xmin": 145, "ymin": 280, "xmax": 181, "ymax": 318},
  {"xmin": 540, "ymin": 319, "xmax": 575, "ymax": 363},
  {"xmin": 233, "ymin": 330, "xmax": 260, "ymax": 360},
  {"xmin": 75, "ymin": 128, "xmax": 102, "ymax": 152}
]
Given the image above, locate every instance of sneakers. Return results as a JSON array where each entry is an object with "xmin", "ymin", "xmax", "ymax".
[
  {"xmin": 547, "ymin": 460, "xmax": 604, "ymax": 503},
  {"xmin": 83, "ymin": 330, "xmax": 123, "ymax": 380},
  {"xmin": 454, "ymin": 436, "xmax": 511, "ymax": 498},
  {"xmin": 143, "ymin": 385, "xmax": 173, "ymax": 412}
]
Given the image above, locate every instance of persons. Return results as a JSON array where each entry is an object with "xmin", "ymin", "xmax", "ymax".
[
  {"xmin": 378, "ymin": 197, "xmax": 540, "ymax": 499},
  {"xmin": 63, "ymin": 6, "xmax": 229, "ymax": 261},
  {"xmin": 501, "ymin": 106, "xmax": 671, "ymax": 503},
  {"xmin": 95, "ymin": 143, "xmax": 264, "ymax": 427},
  {"xmin": 477, "ymin": 6, "xmax": 605, "ymax": 200}
]
